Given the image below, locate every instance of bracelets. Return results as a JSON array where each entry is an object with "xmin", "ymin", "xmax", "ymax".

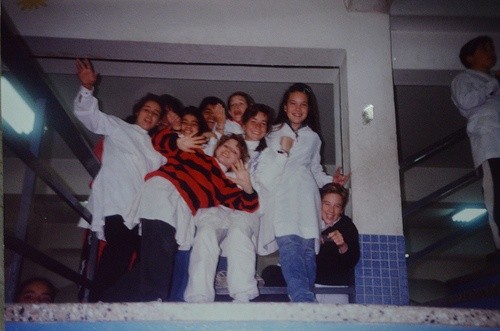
[{"xmin": 281, "ymin": 149, "xmax": 290, "ymax": 154}]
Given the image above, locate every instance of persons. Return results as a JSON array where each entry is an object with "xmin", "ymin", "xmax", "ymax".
[
  {"xmin": 88, "ymin": 92, "xmax": 338, "ymax": 273},
  {"xmin": 73, "ymin": 56, "xmax": 169, "ymax": 302},
  {"xmin": 263, "ymin": 181, "xmax": 361, "ymax": 285},
  {"xmin": 98, "ymin": 130, "xmax": 260, "ymax": 303},
  {"xmin": 264, "ymin": 83, "xmax": 353, "ymax": 303},
  {"xmin": 16, "ymin": 276, "xmax": 56, "ymax": 303},
  {"xmin": 450, "ymin": 35, "xmax": 500, "ymax": 251}
]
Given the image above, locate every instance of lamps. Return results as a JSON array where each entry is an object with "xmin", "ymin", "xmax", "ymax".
[{"xmin": 1, "ymin": 76, "xmax": 50, "ymax": 139}]
